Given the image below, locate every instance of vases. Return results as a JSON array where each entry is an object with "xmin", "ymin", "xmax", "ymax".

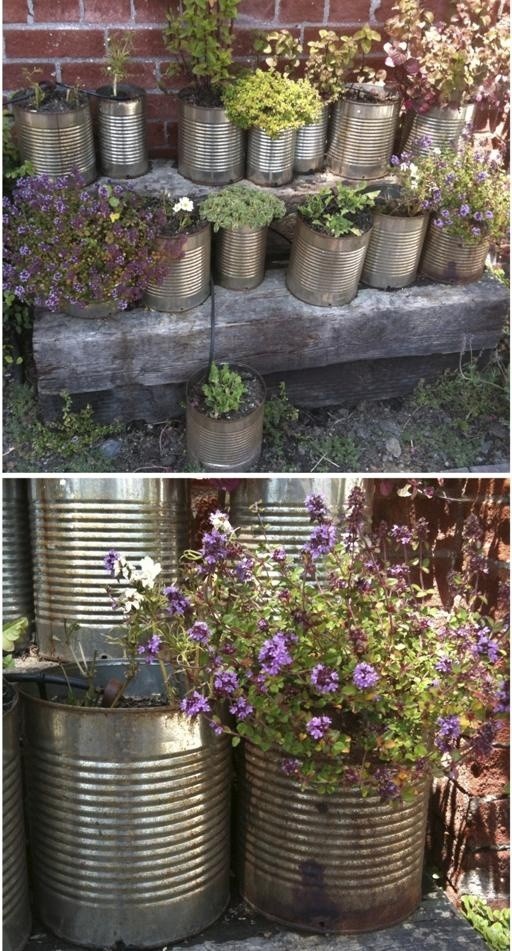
[{"xmin": 419, "ymin": 222, "xmax": 492, "ymax": 286}]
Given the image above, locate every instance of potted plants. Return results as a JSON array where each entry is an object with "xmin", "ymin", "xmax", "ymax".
[{"xmin": 187, "ymin": 361, "xmax": 266, "ymax": 472}]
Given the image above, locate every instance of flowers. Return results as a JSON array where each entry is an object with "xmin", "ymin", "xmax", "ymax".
[
  {"xmin": 4, "ymin": 169, "xmax": 194, "ymax": 316},
  {"xmin": 104, "ymin": 478, "xmax": 509, "ymax": 804},
  {"xmin": 384, "ymin": 0, "xmax": 510, "ymax": 240}
]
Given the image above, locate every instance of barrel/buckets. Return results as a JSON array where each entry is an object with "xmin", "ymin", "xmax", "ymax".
[{"xmin": 2, "ymin": 64, "xmax": 497, "ymax": 949}]
